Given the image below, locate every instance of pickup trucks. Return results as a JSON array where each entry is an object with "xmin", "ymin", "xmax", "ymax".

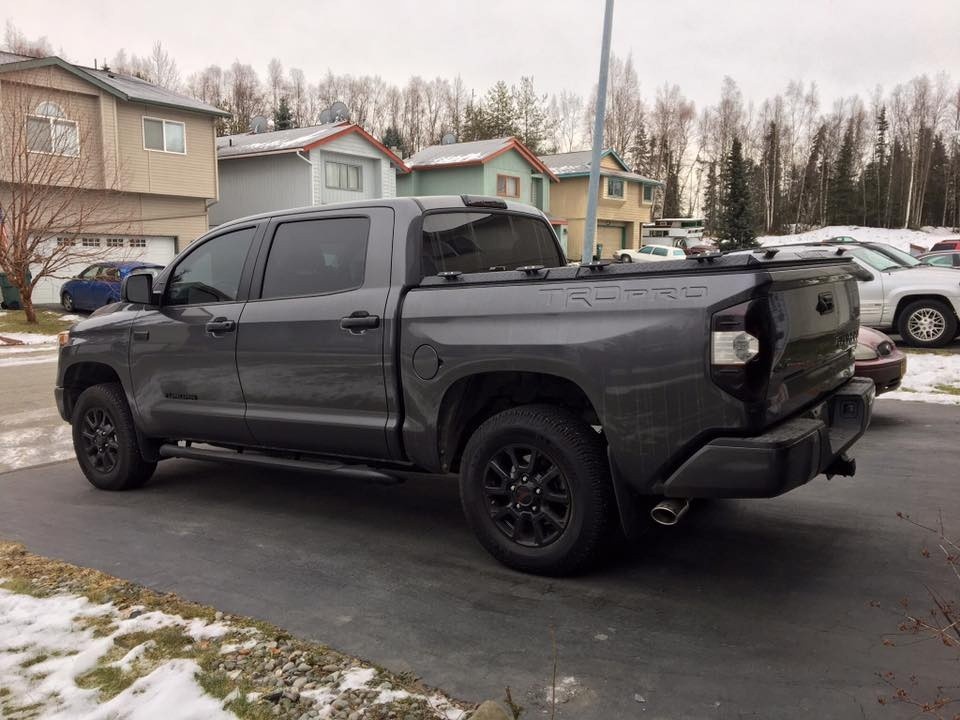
[{"xmin": 54, "ymin": 196, "xmax": 875, "ymax": 575}]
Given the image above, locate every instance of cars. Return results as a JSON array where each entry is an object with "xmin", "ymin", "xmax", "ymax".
[
  {"xmin": 753, "ymin": 236, "xmax": 960, "ymax": 396},
  {"xmin": 60, "ymin": 260, "xmax": 165, "ymax": 312},
  {"xmin": 613, "ymin": 245, "xmax": 687, "ymax": 264}
]
